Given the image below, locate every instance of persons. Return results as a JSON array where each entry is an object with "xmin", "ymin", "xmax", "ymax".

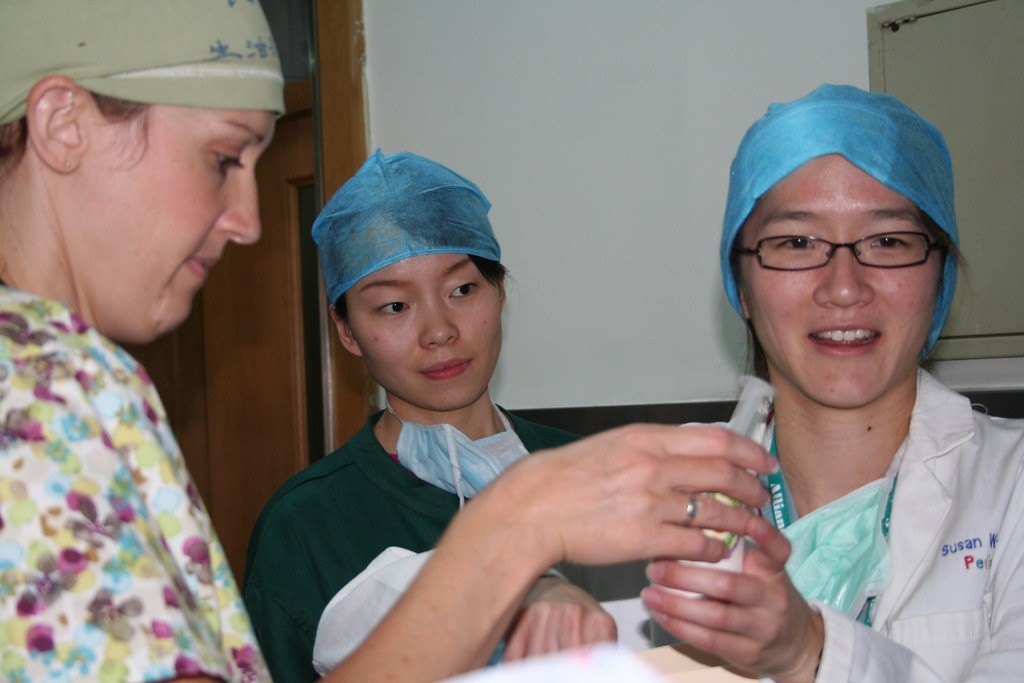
[
  {"xmin": 641, "ymin": 83, "xmax": 1024, "ymax": 683},
  {"xmin": 247, "ymin": 152, "xmax": 618, "ymax": 683},
  {"xmin": 0, "ymin": 0, "xmax": 776, "ymax": 683}
]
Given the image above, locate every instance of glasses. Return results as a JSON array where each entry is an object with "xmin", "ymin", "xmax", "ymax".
[{"xmin": 735, "ymin": 229, "xmax": 947, "ymax": 272}]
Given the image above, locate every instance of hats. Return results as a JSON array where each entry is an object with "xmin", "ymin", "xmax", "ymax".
[{"xmin": 0, "ymin": 0, "xmax": 285, "ymax": 129}]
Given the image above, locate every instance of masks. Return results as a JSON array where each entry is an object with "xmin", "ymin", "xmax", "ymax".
[
  {"xmin": 776, "ymin": 476, "xmax": 894, "ymax": 620},
  {"xmin": 389, "ymin": 409, "xmax": 532, "ymax": 498}
]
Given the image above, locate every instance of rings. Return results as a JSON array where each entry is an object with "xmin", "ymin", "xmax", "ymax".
[{"xmin": 680, "ymin": 492, "xmax": 697, "ymax": 526}]
